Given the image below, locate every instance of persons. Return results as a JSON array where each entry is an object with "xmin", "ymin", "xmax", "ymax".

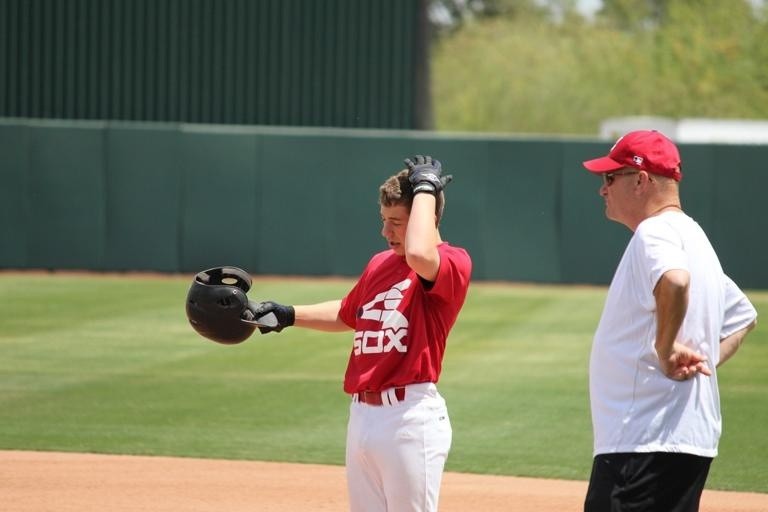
[
  {"xmin": 253, "ymin": 154, "xmax": 474, "ymax": 512},
  {"xmin": 579, "ymin": 129, "xmax": 759, "ymax": 512}
]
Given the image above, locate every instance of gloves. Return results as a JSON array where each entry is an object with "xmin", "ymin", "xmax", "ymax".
[
  {"xmin": 403, "ymin": 154, "xmax": 453, "ymax": 196},
  {"xmin": 256, "ymin": 301, "xmax": 295, "ymax": 335}
]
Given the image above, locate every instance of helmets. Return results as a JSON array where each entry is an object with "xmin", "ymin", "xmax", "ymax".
[{"xmin": 186, "ymin": 266, "xmax": 279, "ymax": 345}]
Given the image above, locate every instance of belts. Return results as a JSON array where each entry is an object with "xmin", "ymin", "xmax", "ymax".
[{"xmin": 359, "ymin": 388, "xmax": 405, "ymax": 406}]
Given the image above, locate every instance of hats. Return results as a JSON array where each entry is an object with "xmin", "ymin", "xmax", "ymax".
[{"xmin": 582, "ymin": 129, "xmax": 682, "ymax": 182}]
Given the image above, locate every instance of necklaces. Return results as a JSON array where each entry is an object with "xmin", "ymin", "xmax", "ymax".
[{"xmin": 645, "ymin": 204, "xmax": 682, "ymax": 218}]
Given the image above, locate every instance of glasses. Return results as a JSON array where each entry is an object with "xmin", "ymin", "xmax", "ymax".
[{"xmin": 605, "ymin": 171, "xmax": 652, "ymax": 186}]
